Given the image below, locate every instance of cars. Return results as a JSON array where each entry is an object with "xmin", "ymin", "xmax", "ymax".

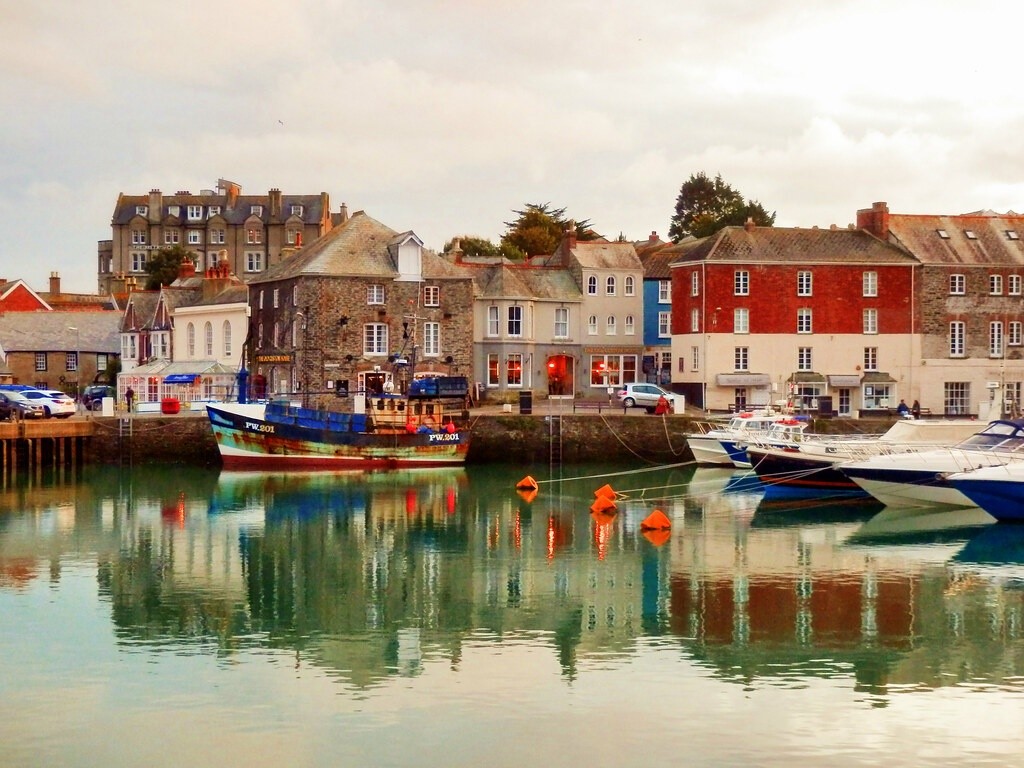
[{"xmin": 0, "ymin": 384, "xmax": 77, "ymax": 419}]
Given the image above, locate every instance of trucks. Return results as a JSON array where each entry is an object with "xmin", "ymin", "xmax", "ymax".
[{"xmin": 403, "ymin": 372, "xmax": 469, "ymax": 408}]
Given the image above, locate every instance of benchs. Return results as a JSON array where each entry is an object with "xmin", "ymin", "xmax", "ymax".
[
  {"xmin": 573, "ymin": 401, "xmax": 628, "ymax": 415},
  {"xmin": 888, "ymin": 408, "xmax": 932, "ymax": 419},
  {"xmin": 728, "ymin": 404, "xmax": 782, "ymax": 413}
]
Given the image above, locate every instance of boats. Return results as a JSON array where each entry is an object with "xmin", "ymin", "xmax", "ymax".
[
  {"xmin": 945, "ymin": 522, "xmax": 1024, "ymax": 583},
  {"xmin": 748, "ymin": 487, "xmax": 886, "ymax": 528},
  {"xmin": 719, "ymin": 467, "xmax": 767, "ymax": 496},
  {"xmin": 840, "ymin": 505, "xmax": 998, "ymax": 547},
  {"xmin": 680, "ymin": 383, "xmax": 1024, "ymax": 522},
  {"xmin": 205, "ymin": 326, "xmax": 478, "ymax": 473},
  {"xmin": 684, "ymin": 465, "xmax": 758, "ymax": 505}
]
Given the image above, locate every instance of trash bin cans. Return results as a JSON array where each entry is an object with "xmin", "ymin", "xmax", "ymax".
[
  {"xmin": 818, "ymin": 396, "xmax": 833, "ymax": 418},
  {"xmin": 519, "ymin": 391, "xmax": 531, "ymax": 413}
]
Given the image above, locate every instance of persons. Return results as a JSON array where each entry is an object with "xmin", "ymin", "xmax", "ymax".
[
  {"xmin": 125, "ymin": 387, "xmax": 134, "ymax": 413},
  {"xmin": 406, "ymin": 417, "xmax": 455, "ymax": 434},
  {"xmin": 896, "ymin": 399, "xmax": 920, "ymax": 419}
]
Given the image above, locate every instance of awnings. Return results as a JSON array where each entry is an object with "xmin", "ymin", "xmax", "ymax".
[{"xmin": 162, "ymin": 374, "xmax": 201, "ymax": 385}]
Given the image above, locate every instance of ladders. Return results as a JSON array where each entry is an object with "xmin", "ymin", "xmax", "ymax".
[
  {"xmin": 550, "ymin": 396, "xmax": 563, "ymax": 463},
  {"xmin": 119, "ymin": 398, "xmax": 133, "ymax": 436}
]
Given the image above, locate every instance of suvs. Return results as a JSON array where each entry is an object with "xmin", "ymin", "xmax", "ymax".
[
  {"xmin": 615, "ymin": 382, "xmax": 680, "ymax": 411},
  {"xmin": 82, "ymin": 381, "xmax": 117, "ymax": 411}
]
